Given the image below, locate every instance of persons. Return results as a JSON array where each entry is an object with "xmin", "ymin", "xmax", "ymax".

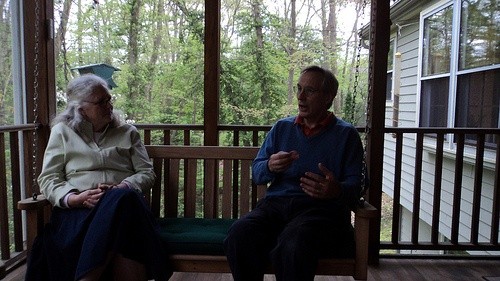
[
  {"xmin": 228, "ymin": 66, "xmax": 365, "ymax": 281},
  {"xmin": 27, "ymin": 74, "xmax": 158, "ymax": 281}
]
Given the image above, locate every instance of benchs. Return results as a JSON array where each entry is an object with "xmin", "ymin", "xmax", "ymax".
[{"xmin": 17, "ymin": 146, "xmax": 378, "ymax": 281}]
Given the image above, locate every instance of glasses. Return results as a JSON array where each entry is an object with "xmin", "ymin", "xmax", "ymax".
[
  {"xmin": 83, "ymin": 97, "xmax": 112, "ymax": 105},
  {"xmin": 293, "ymin": 85, "xmax": 316, "ymax": 97}
]
